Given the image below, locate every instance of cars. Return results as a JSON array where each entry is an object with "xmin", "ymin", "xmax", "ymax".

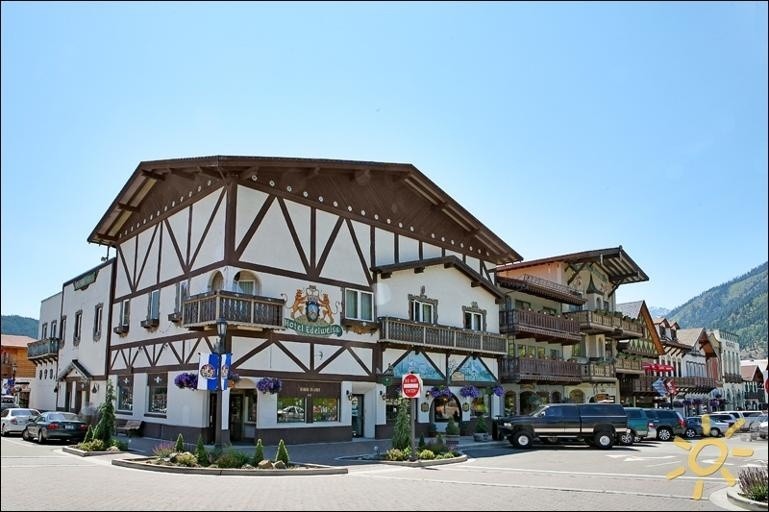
[
  {"xmin": 22, "ymin": 411, "xmax": 87, "ymax": 443},
  {"xmin": 751, "ymin": 414, "xmax": 769, "ymax": 439},
  {"xmin": 684, "ymin": 414, "xmax": 737, "ymax": 437},
  {"xmin": 1, "ymin": 407, "xmax": 42, "ymax": 436}
]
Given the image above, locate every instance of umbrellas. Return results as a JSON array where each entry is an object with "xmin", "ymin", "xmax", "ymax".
[{"xmin": 642, "ymin": 363, "xmax": 673, "ymax": 371}]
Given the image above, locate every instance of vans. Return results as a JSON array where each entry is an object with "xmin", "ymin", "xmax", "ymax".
[
  {"xmin": 713, "ymin": 411, "xmax": 764, "ymax": 431},
  {"xmin": 500, "ymin": 400, "xmax": 687, "ymax": 447}
]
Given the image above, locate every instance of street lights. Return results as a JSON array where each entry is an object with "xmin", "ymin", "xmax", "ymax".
[
  {"xmin": 11, "ymin": 362, "xmax": 18, "ymax": 385},
  {"xmin": 215, "ymin": 317, "xmax": 228, "ymax": 451}
]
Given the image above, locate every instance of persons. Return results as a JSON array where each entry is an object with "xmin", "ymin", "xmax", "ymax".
[{"xmin": 352, "ymin": 397, "xmax": 360, "ymax": 438}]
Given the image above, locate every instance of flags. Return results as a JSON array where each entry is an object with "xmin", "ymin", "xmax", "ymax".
[
  {"xmin": 220, "ymin": 353, "xmax": 231, "ymax": 390},
  {"xmin": 667, "ymin": 381, "xmax": 677, "ymax": 395},
  {"xmin": 197, "ymin": 352, "xmax": 219, "ymax": 391},
  {"xmin": 652, "ymin": 379, "xmax": 667, "ymax": 397}
]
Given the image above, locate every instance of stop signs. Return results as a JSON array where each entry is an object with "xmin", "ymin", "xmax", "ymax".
[{"xmin": 401, "ymin": 372, "xmax": 422, "ymax": 400}]
